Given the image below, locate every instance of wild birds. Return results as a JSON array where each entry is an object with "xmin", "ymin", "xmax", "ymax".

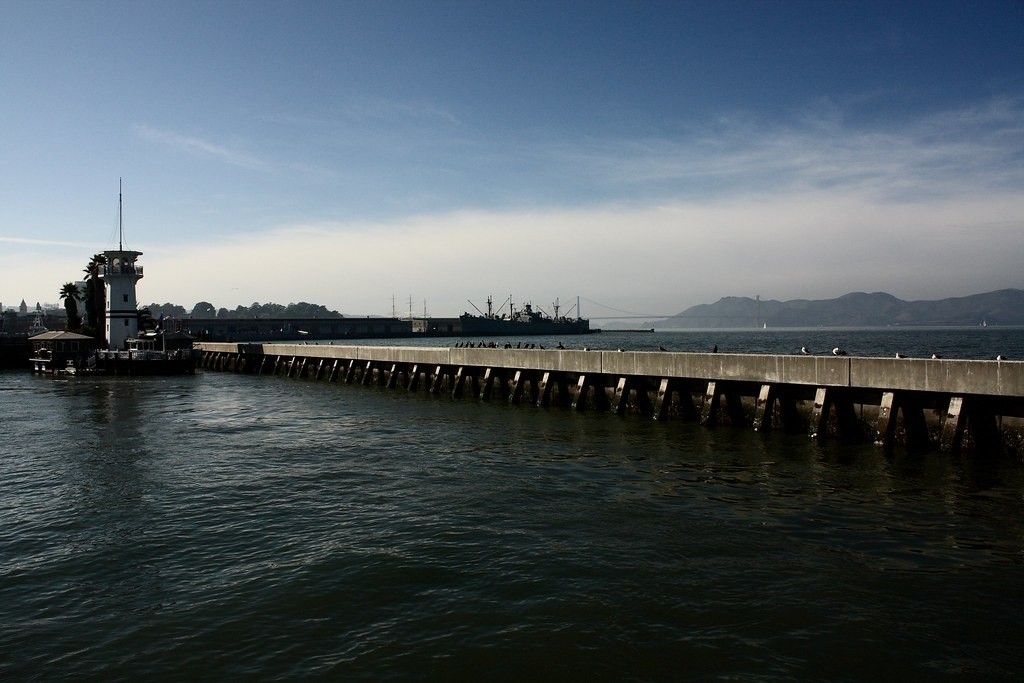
[
  {"xmin": 895, "ymin": 352, "xmax": 909, "ymax": 359},
  {"xmin": 454, "ymin": 339, "xmax": 545, "ymax": 352},
  {"xmin": 801, "ymin": 344, "xmax": 811, "ymax": 356},
  {"xmin": 931, "ymin": 353, "xmax": 943, "ymax": 359},
  {"xmin": 555, "ymin": 342, "xmax": 565, "ymax": 350},
  {"xmin": 997, "ymin": 355, "xmax": 1009, "ymax": 361},
  {"xmin": 299, "ymin": 341, "xmax": 333, "ymax": 345},
  {"xmin": 617, "ymin": 347, "xmax": 628, "ymax": 352},
  {"xmin": 832, "ymin": 345, "xmax": 848, "ymax": 356},
  {"xmin": 584, "ymin": 346, "xmax": 591, "ymax": 352},
  {"xmin": 713, "ymin": 345, "xmax": 718, "ymax": 353},
  {"xmin": 659, "ymin": 345, "xmax": 668, "ymax": 353}
]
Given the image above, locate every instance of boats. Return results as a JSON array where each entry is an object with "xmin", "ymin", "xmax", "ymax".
[{"xmin": 454, "ymin": 294, "xmax": 590, "ymax": 337}]
